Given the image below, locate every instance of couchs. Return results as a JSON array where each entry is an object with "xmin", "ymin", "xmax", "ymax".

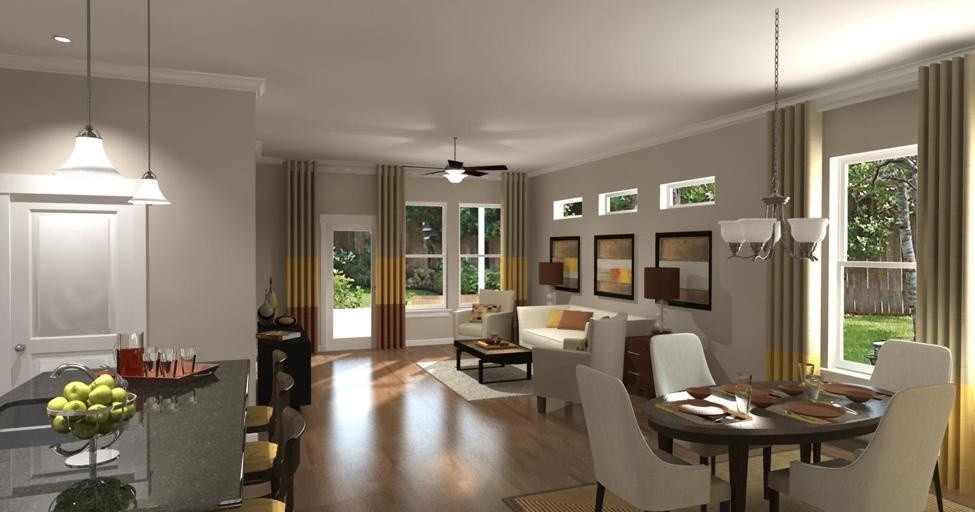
[
  {"xmin": 452, "ymin": 289, "xmax": 515, "ymax": 343},
  {"xmin": 533, "ymin": 311, "xmax": 629, "ymax": 414},
  {"xmin": 517, "ymin": 305, "xmax": 656, "ymax": 351}
]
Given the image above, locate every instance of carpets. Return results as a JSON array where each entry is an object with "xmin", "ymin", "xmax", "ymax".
[
  {"xmin": 416, "ymin": 358, "xmax": 534, "ymax": 402},
  {"xmin": 502, "ymin": 446, "xmax": 973, "ymax": 510}
]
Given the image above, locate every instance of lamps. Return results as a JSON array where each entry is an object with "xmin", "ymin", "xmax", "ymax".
[
  {"xmin": 719, "ymin": 7, "xmax": 830, "ymax": 263},
  {"xmin": 644, "ymin": 268, "xmax": 681, "ymax": 333},
  {"xmin": 538, "ymin": 262, "xmax": 564, "ymax": 306}
]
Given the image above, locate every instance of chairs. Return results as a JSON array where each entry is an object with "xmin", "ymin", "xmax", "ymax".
[
  {"xmin": 767, "ymin": 382, "xmax": 957, "ymax": 512},
  {"xmin": 246, "ymin": 349, "xmax": 287, "ymax": 440},
  {"xmin": 223, "ymin": 407, "xmax": 306, "ymax": 511},
  {"xmin": 244, "ymin": 371, "xmax": 295, "ymax": 496},
  {"xmin": 854, "ymin": 339, "xmax": 953, "ymax": 511},
  {"xmin": 650, "ymin": 331, "xmax": 772, "ymax": 500},
  {"xmin": 576, "ymin": 364, "xmax": 711, "ymax": 512}
]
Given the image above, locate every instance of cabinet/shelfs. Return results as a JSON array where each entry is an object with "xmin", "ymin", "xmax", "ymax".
[{"xmin": 257, "ymin": 321, "xmax": 313, "ymax": 408}]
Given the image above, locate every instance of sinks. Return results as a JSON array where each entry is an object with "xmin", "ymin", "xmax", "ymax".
[{"xmin": 0, "ymin": 395, "xmax": 83, "ymax": 454}]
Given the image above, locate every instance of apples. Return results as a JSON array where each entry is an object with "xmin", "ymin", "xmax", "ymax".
[
  {"xmin": 127, "ymin": 401, "xmax": 136, "ymax": 417},
  {"xmin": 89, "ymin": 373, "xmax": 114, "ymax": 391},
  {"xmin": 112, "ymin": 387, "xmax": 128, "ymax": 403},
  {"xmin": 86, "ymin": 404, "xmax": 110, "ymax": 423},
  {"xmin": 53, "ymin": 414, "xmax": 68, "ymax": 433},
  {"xmin": 48, "ymin": 396, "xmax": 67, "ymax": 410},
  {"xmin": 111, "ymin": 402, "xmax": 128, "ymax": 420},
  {"xmin": 74, "ymin": 419, "xmax": 98, "ymax": 438},
  {"xmin": 63, "ymin": 381, "xmax": 89, "ymax": 401},
  {"xmin": 62, "ymin": 399, "xmax": 86, "ymax": 421},
  {"xmin": 88, "ymin": 384, "xmax": 112, "ymax": 404}
]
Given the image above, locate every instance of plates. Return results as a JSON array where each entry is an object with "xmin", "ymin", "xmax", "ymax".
[
  {"xmin": 671, "ymin": 399, "xmax": 729, "ymax": 416},
  {"xmin": 823, "ymin": 384, "xmax": 873, "ymax": 396},
  {"xmin": 784, "ymin": 401, "xmax": 846, "ymax": 419},
  {"xmin": 95, "ymin": 362, "xmax": 220, "ymax": 387},
  {"xmin": 720, "ymin": 383, "xmax": 772, "ymax": 396}
]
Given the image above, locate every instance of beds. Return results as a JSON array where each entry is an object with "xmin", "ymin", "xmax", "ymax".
[
  {"xmin": 128, "ymin": 1, "xmax": 171, "ymax": 207},
  {"xmin": 443, "ymin": 136, "xmax": 469, "ymax": 184},
  {"xmin": 53, "ymin": 1, "xmax": 124, "ymax": 188}
]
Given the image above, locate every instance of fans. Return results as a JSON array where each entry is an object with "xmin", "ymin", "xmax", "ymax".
[{"xmin": 402, "ymin": 159, "xmax": 508, "ymax": 177}]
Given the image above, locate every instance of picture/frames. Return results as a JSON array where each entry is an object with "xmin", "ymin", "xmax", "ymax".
[
  {"xmin": 549, "ymin": 235, "xmax": 581, "ymax": 294},
  {"xmin": 593, "ymin": 234, "xmax": 635, "ymax": 300},
  {"xmin": 655, "ymin": 231, "xmax": 713, "ymax": 311}
]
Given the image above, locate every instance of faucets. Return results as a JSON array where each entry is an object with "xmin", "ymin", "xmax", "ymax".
[{"xmin": 49, "ymin": 363, "xmax": 95, "ymax": 384}]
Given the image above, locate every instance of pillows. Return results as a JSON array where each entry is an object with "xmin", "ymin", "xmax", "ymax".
[
  {"xmin": 546, "ymin": 308, "xmax": 580, "ymax": 328},
  {"xmin": 576, "ymin": 316, "xmax": 609, "ymax": 351},
  {"xmin": 557, "ymin": 310, "xmax": 593, "ymax": 331},
  {"xmin": 469, "ymin": 303, "xmax": 500, "ymax": 323}
]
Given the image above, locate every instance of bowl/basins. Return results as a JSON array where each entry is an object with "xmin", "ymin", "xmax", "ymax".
[
  {"xmin": 751, "ymin": 395, "xmax": 776, "ymax": 408},
  {"xmin": 687, "ymin": 388, "xmax": 713, "ymax": 399},
  {"xmin": 778, "ymin": 384, "xmax": 806, "ymax": 396},
  {"xmin": 844, "ymin": 390, "xmax": 873, "ymax": 403}
]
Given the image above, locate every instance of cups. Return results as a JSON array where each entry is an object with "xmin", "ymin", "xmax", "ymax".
[
  {"xmin": 144, "ymin": 345, "xmax": 157, "ymax": 374},
  {"xmin": 180, "ymin": 344, "xmax": 197, "ymax": 376},
  {"xmin": 735, "ymin": 384, "xmax": 752, "ymax": 416},
  {"xmin": 112, "ymin": 331, "xmax": 144, "ymax": 376},
  {"xmin": 735, "ymin": 371, "xmax": 752, "ymax": 386},
  {"xmin": 143, "ymin": 352, "xmax": 160, "ymax": 377},
  {"xmin": 159, "ymin": 351, "xmax": 178, "ymax": 378},
  {"xmin": 805, "ymin": 375, "xmax": 821, "ymax": 402},
  {"xmin": 156, "ymin": 344, "xmax": 174, "ymax": 376}
]
